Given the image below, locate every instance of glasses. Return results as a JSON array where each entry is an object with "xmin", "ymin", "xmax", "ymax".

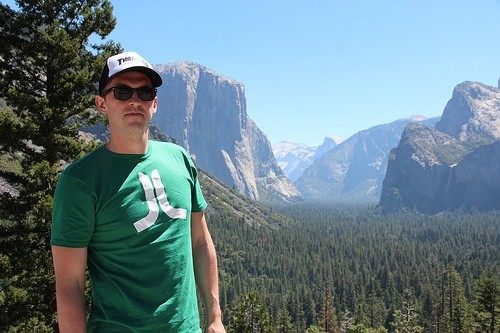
[{"xmin": 100, "ymin": 86, "xmax": 158, "ymax": 102}]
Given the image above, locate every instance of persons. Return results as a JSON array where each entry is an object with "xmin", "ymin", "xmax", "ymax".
[{"xmin": 52, "ymin": 51, "xmax": 227, "ymax": 333}]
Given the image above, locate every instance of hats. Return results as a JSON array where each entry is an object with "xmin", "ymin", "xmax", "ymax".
[{"xmin": 99, "ymin": 51, "xmax": 163, "ymax": 95}]
